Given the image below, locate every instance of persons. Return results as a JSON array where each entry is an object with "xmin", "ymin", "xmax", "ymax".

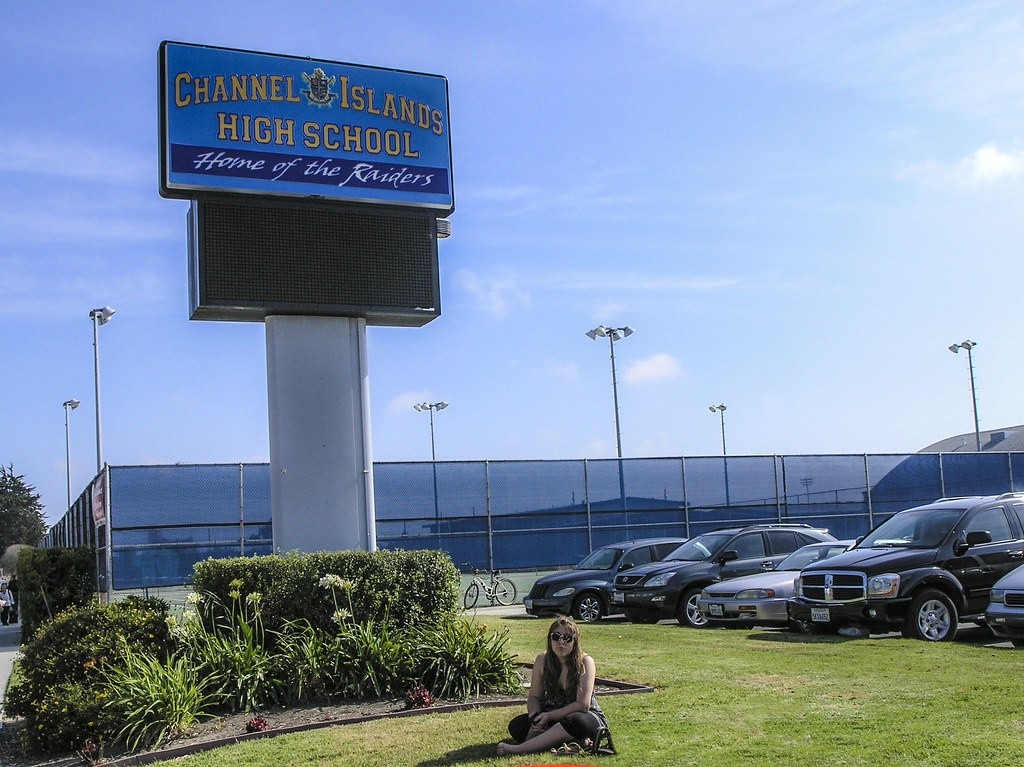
[
  {"xmin": 496, "ymin": 616, "xmax": 609, "ymax": 755},
  {"xmin": 0, "ymin": 580, "xmax": 15, "ymax": 626},
  {"xmin": 10, "ymin": 575, "xmax": 19, "ymax": 623}
]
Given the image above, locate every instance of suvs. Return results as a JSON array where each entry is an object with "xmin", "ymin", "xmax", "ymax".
[
  {"xmin": 786, "ymin": 491, "xmax": 1024, "ymax": 642},
  {"xmin": 612, "ymin": 523, "xmax": 840, "ymax": 628}
]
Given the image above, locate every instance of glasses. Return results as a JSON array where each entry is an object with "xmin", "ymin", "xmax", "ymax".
[{"xmin": 550, "ymin": 632, "xmax": 575, "ymax": 643}]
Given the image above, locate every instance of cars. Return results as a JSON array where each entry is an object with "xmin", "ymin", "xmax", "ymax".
[
  {"xmin": 523, "ymin": 536, "xmax": 710, "ymax": 625},
  {"xmin": 696, "ymin": 537, "xmax": 911, "ymax": 634},
  {"xmin": 984, "ymin": 561, "xmax": 1024, "ymax": 648}
]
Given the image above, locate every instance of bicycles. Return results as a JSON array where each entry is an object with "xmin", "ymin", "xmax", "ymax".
[{"xmin": 460, "ymin": 560, "xmax": 517, "ymax": 610}]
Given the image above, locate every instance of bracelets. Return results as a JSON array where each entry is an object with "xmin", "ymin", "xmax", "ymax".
[{"xmin": 548, "ymin": 711, "xmax": 551, "ymax": 721}]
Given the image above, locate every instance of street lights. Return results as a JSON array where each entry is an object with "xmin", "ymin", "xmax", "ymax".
[
  {"xmin": 62, "ymin": 399, "xmax": 82, "ymax": 546},
  {"xmin": 90, "ymin": 306, "xmax": 116, "ymax": 610},
  {"xmin": 412, "ymin": 401, "xmax": 451, "ymax": 552},
  {"xmin": 800, "ymin": 476, "xmax": 815, "ymax": 515},
  {"xmin": 950, "ymin": 339, "xmax": 987, "ymax": 494},
  {"xmin": 585, "ymin": 324, "xmax": 636, "ymax": 541},
  {"xmin": 708, "ymin": 403, "xmax": 735, "ymax": 509}
]
